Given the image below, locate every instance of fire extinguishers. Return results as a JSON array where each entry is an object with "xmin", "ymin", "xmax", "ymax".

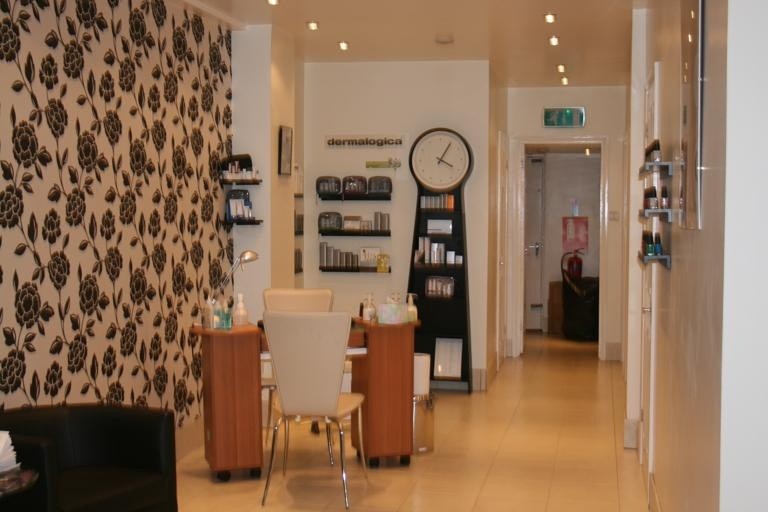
[{"xmin": 561, "ymin": 248, "xmax": 585, "ymax": 281}]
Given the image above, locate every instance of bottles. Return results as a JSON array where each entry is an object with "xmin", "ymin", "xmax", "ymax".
[
  {"xmin": 642, "ymin": 230, "xmax": 662, "ymax": 256},
  {"xmin": 221, "ymin": 300, "xmax": 232, "ymax": 328},
  {"xmin": 212, "ymin": 301, "xmax": 222, "ymax": 328},
  {"xmin": 644, "ymin": 186, "xmax": 669, "ymax": 208},
  {"xmin": 644, "ymin": 139, "xmax": 661, "ymax": 162}
]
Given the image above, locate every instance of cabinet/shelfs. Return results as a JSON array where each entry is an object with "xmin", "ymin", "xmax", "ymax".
[
  {"xmin": 406, "ymin": 191, "xmax": 478, "ymax": 394},
  {"xmin": 218, "ymin": 178, "xmax": 264, "ymax": 225},
  {"xmin": 319, "ymin": 192, "xmax": 394, "ymax": 274},
  {"xmin": 636, "ymin": 161, "xmax": 674, "ymax": 270}
]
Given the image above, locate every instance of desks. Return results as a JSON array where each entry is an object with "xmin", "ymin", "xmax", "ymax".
[
  {"xmin": 191, "ymin": 312, "xmax": 422, "ymax": 480},
  {"xmin": 0, "ymin": 461, "xmax": 49, "ymax": 512}
]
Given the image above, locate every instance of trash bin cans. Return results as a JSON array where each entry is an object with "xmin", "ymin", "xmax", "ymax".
[{"xmin": 413, "ymin": 393, "xmax": 437, "ymax": 455}]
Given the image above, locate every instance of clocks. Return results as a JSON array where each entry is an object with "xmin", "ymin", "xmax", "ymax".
[{"xmin": 410, "ymin": 126, "xmax": 475, "ymax": 194}]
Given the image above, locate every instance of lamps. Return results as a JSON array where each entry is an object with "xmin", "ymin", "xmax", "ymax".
[{"xmin": 209, "ymin": 249, "xmax": 259, "ymax": 301}]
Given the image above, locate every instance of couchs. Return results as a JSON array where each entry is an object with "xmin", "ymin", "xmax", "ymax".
[{"xmin": 1, "ymin": 402, "xmax": 179, "ymax": 512}]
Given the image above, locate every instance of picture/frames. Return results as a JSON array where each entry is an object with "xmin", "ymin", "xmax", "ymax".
[
  {"xmin": 277, "ymin": 125, "xmax": 293, "ymax": 178},
  {"xmin": 642, "ymin": 62, "xmax": 660, "ymax": 150}
]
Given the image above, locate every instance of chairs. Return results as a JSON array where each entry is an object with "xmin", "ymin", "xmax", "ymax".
[
  {"xmin": 265, "ymin": 286, "xmax": 336, "ymax": 466},
  {"xmin": 253, "ymin": 310, "xmax": 375, "ymax": 510}
]
